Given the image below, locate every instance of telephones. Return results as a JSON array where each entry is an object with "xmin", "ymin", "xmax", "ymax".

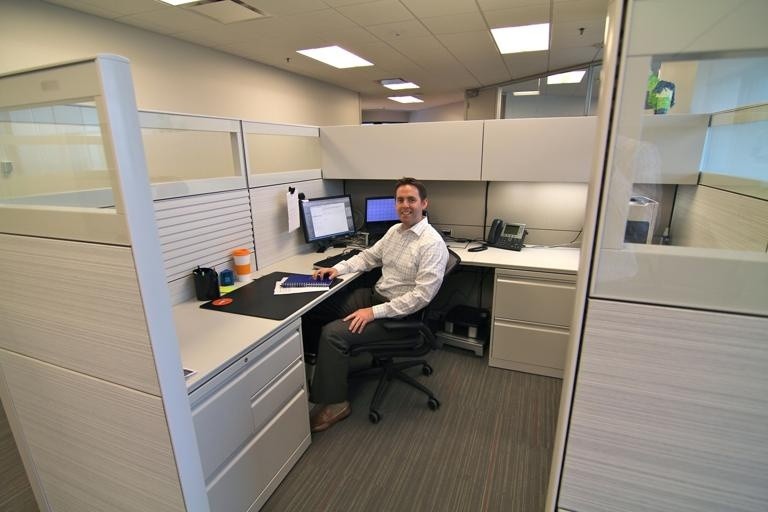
[{"xmin": 487, "ymin": 219, "xmax": 527, "ymax": 251}]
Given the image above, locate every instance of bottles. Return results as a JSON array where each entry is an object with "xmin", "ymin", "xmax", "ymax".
[{"xmin": 660, "ymin": 226, "xmax": 669, "ymax": 244}]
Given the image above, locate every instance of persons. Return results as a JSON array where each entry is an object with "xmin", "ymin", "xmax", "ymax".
[{"xmin": 308, "ymin": 178, "xmax": 449, "ymax": 433}]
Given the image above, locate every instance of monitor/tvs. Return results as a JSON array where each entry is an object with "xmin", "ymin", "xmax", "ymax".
[{"xmin": 299, "ymin": 194, "xmax": 357, "ymax": 252}]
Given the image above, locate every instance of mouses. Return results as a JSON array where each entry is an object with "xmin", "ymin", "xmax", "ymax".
[{"xmin": 315, "ymin": 273, "xmax": 329, "ymax": 281}]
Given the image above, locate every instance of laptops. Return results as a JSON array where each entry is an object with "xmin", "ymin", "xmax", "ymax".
[{"xmin": 365, "ymin": 196, "xmax": 402, "ymax": 246}]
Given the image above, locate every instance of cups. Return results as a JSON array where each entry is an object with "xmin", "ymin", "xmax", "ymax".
[{"xmin": 231, "ymin": 250, "xmax": 251, "ymax": 281}]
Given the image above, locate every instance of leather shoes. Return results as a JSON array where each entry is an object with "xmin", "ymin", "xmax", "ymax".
[{"xmin": 309, "ymin": 400, "xmax": 352, "ymax": 433}]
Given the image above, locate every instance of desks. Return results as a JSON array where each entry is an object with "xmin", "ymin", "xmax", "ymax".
[{"xmin": 170, "ymin": 240, "xmax": 582, "ymax": 510}]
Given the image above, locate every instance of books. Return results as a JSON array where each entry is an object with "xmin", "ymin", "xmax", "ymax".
[{"xmin": 280, "ymin": 274, "xmax": 335, "ymax": 287}]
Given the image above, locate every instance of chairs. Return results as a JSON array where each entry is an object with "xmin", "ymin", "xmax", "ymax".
[{"xmin": 350, "ymin": 248, "xmax": 461, "ymax": 424}]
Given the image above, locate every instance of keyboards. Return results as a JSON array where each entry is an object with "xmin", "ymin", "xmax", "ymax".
[{"xmin": 313, "ymin": 249, "xmax": 362, "ymax": 268}]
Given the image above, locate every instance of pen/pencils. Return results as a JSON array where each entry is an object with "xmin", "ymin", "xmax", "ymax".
[{"xmin": 194, "ymin": 265, "xmax": 216, "ymax": 276}]
